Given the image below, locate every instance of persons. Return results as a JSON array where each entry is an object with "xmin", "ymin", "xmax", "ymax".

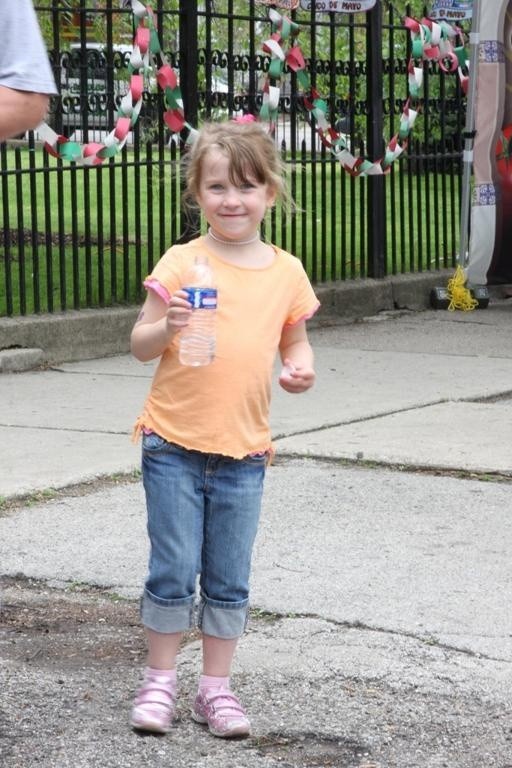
[
  {"xmin": 127, "ymin": 116, "xmax": 320, "ymax": 741},
  {"xmin": 0, "ymin": 0, "xmax": 62, "ymax": 146}
]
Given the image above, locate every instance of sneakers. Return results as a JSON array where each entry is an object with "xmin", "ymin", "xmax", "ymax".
[
  {"xmin": 189, "ymin": 685, "xmax": 251, "ymax": 737},
  {"xmin": 129, "ymin": 675, "xmax": 178, "ymax": 732}
]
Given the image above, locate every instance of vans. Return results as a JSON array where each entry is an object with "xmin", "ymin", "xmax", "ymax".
[{"xmin": 60, "ymin": 39, "xmax": 245, "ymax": 146}]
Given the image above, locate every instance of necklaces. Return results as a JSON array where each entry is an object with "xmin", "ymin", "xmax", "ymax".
[{"xmin": 208, "ymin": 226, "xmax": 260, "ymax": 247}]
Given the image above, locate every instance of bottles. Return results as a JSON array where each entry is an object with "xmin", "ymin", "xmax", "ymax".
[{"xmin": 177, "ymin": 253, "xmax": 221, "ymax": 369}]
